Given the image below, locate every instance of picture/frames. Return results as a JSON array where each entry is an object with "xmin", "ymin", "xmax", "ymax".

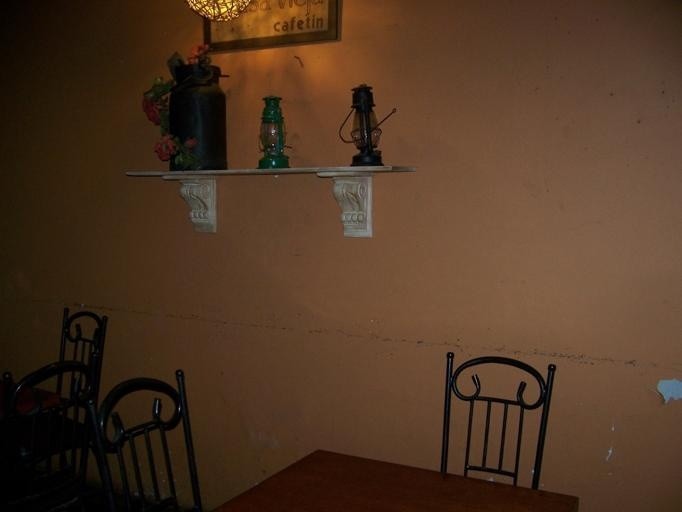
[{"xmin": 202, "ymin": 0, "xmax": 342, "ymax": 53}]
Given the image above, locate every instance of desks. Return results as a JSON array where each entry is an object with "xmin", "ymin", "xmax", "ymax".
[{"xmin": 214, "ymin": 450, "xmax": 578, "ymax": 512}]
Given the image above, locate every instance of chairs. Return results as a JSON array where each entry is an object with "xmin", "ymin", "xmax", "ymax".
[
  {"xmin": 0, "ymin": 306, "xmax": 108, "ymax": 512},
  {"xmin": 441, "ymin": 351, "xmax": 555, "ymax": 489},
  {"xmin": 88, "ymin": 369, "xmax": 201, "ymax": 512}
]
